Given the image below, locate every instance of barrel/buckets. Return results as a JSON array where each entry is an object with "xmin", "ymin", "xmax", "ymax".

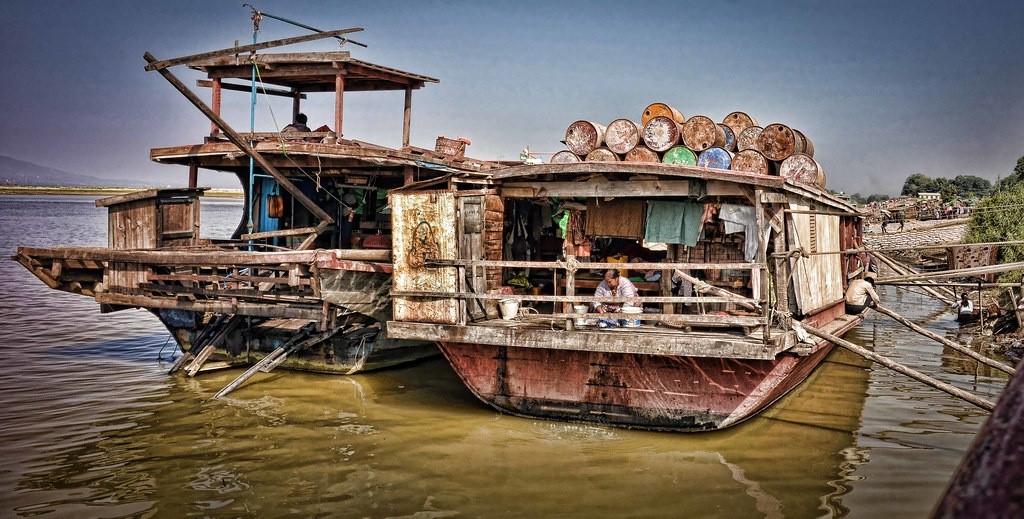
[
  {"xmin": 621, "ymin": 306, "xmax": 643, "ymax": 327},
  {"xmin": 571, "ymin": 305, "xmax": 589, "ymax": 324},
  {"xmin": 549, "ymin": 103, "xmax": 826, "ymax": 189},
  {"xmin": 435, "ymin": 138, "xmax": 465, "ymax": 157},
  {"xmin": 498, "ymin": 300, "xmax": 520, "ymax": 319}
]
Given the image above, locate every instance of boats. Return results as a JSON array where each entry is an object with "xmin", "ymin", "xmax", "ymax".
[{"xmin": 11, "ymin": 3, "xmax": 875, "ymax": 434}]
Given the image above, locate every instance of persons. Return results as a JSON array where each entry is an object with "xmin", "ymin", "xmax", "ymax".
[
  {"xmin": 952, "ymin": 292, "xmax": 974, "ymax": 327},
  {"xmin": 843, "ymin": 276, "xmax": 880, "ymax": 315},
  {"xmin": 881, "ymin": 217, "xmax": 888, "ymax": 232},
  {"xmin": 897, "ymin": 220, "xmax": 905, "ymax": 232},
  {"xmin": 593, "ymin": 269, "xmax": 642, "ymax": 313},
  {"xmin": 934, "ymin": 207, "xmax": 941, "ymax": 219},
  {"xmin": 284, "ymin": 113, "xmax": 312, "ymax": 141},
  {"xmin": 945, "ymin": 203, "xmax": 954, "ymax": 219}
]
